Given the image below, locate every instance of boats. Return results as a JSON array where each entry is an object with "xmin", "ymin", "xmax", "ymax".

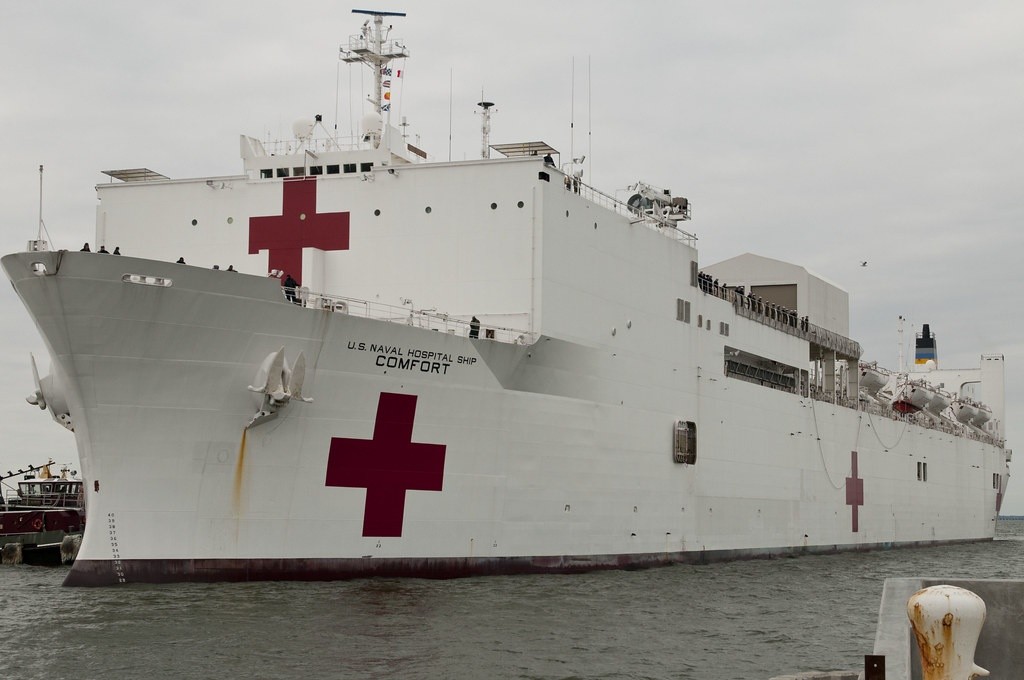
[{"xmin": 0, "ymin": 460, "xmax": 85, "ymax": 567}]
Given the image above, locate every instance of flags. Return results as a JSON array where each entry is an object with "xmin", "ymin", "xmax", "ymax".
[
  {"xmin": 383, "ymin": 92, "xmax": 390, "ymax": 100},
  {"xmin": 397, "ymin": 70, "xmax": 403, "ymax": 78},
  {"xmin": 384, "ymin": 68, "xmax": 392, "ymax": 75},
  {"xmin": 382, "ymin": 104, "xmax": 389, "ymax": 111},
  {"xmin": 382, "ymin": 80, "xmax": 391, "ymax": 87}
]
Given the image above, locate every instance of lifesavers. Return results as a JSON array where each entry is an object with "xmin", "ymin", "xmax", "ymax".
[
  {"xmin": 34, "ymin": 519, "xmax": 43, "ymax": 529},
  {"xmin": 626, "ymin": 193, "xmax": 646, "ymax": 213}
]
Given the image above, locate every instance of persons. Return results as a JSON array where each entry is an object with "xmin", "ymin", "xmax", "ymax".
[
  {"xmin": 532, "ymin": 150, "xmax": 556, "ymax": 168},
  {"xmin": 735, "ymin": 286, "xmax": 744, "ymax": 306},
  {"xmin": 284, "ymin": 275, "xmax": 299, "ymax": 304},
  {"xmin": 801, "ymin": 317, "xmax": 804, "ymax": 331},
  {"xmin": 698, "ymin": 271, "xmax": 719, "ymax": 297},
  {"xmin": 470, "ymin": 316, "xmax": 480, "ymax": 338},
  {"xmin": 805, "ymin": 316, "xmax": 808, "ymax": 332},
  {"xmin": 722, "ymin": 283, "xmax": 727, "ymax": 299},
  {"xmin": 226, "ymin": 265, "xmax": 237, "ymax": 272},
  {"xmin": 98, "ymin": 246, "xmax": 109, "ymax": 253},
  {"xmin": 789, "ymin": 310, "xmax": 797, "ymax": 328},
  {"xmin": 113, "ymin": 247, "xmax": 120, "ymax": 255},
  {"xmin": 177, "ymin": 257, "xmax": 185, "ymax": 264},
  {"xmin": 80, "ymin": 243, "xmax": 91, "ymax": 252},
  {"xmin": 747, "ymin": 293, "xmax": 788, "ymax": 324}
]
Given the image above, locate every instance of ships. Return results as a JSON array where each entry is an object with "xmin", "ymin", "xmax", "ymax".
[{"xmin": 0, "ymin": 10, "xmax": 1013, "ymax": 588}]
